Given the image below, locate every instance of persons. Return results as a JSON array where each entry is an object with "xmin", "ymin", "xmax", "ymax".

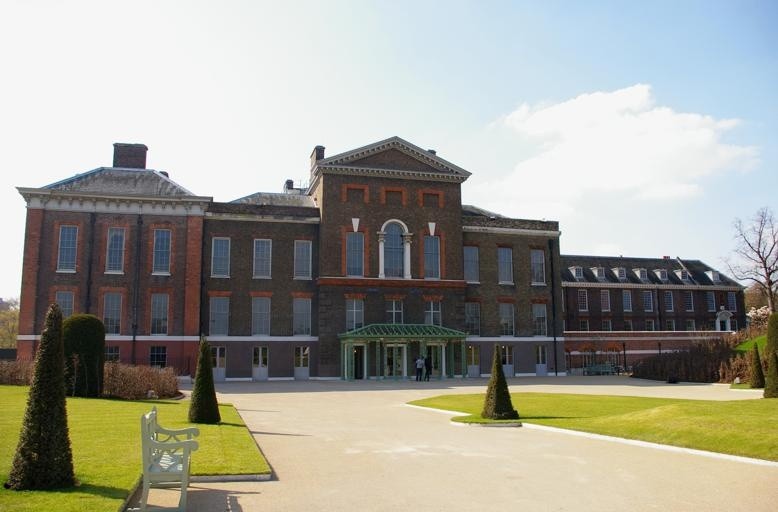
[
  {"xmin": 424, "ymin": 357, "xmax": 431, "ymax": 381},
  {"xmin": 415, "ymin": 353, "xmax": 424, "ymax": 381}
]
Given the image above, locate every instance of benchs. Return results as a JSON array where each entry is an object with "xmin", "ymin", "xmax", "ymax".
[{"xmin": 138, "ymin": 405, "xmax": 199, "ymax": 511}]
